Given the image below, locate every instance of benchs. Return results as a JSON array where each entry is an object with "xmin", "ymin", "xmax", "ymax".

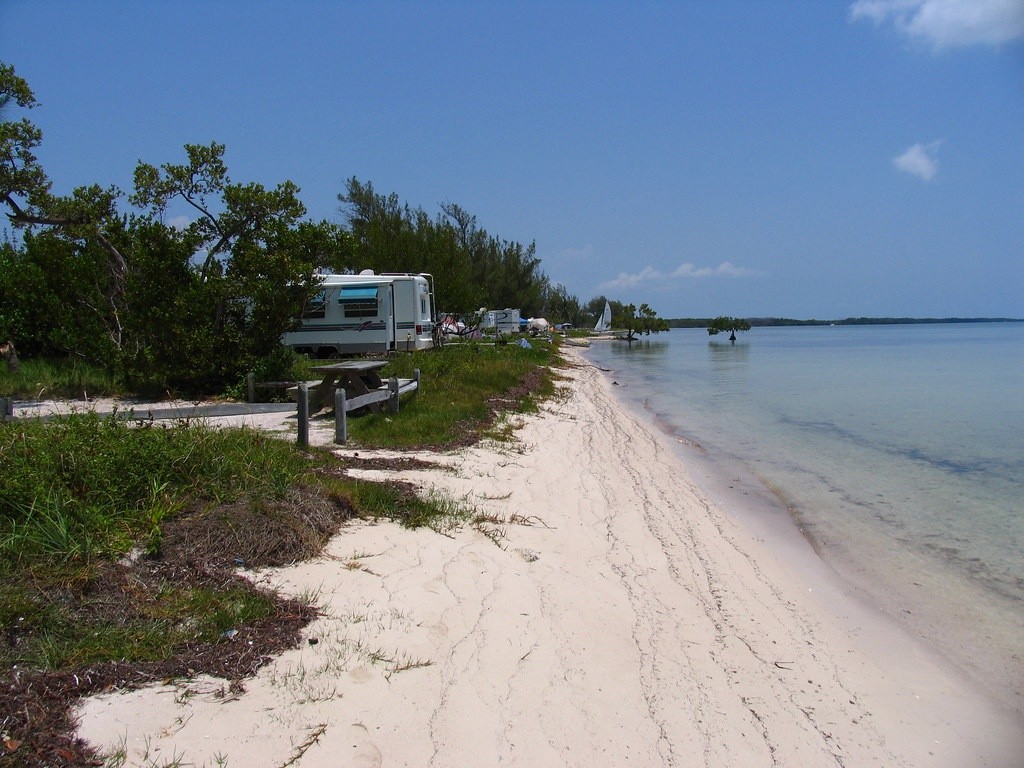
[{"xmin": 287, "ymin": 378, "xmax": 415, "ymax": 416}]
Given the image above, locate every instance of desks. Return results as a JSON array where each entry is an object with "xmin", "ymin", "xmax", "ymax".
[{"xmin": 308, "ymin": 360, "xmax": 389, "ymax": 419}]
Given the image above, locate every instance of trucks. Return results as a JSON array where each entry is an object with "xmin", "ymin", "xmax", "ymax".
[
  {"xmin": 357, "ymin": 269, "xmax": 438, "ymax": 353},
  {"xmin": 223, "ymin": 268, "xmax": 399, "ymax": 362}
]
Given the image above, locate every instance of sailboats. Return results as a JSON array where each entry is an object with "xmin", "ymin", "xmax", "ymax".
[{"xmin": 594, "ymin": 301, "xmax": 612, "ymax": 332}]
